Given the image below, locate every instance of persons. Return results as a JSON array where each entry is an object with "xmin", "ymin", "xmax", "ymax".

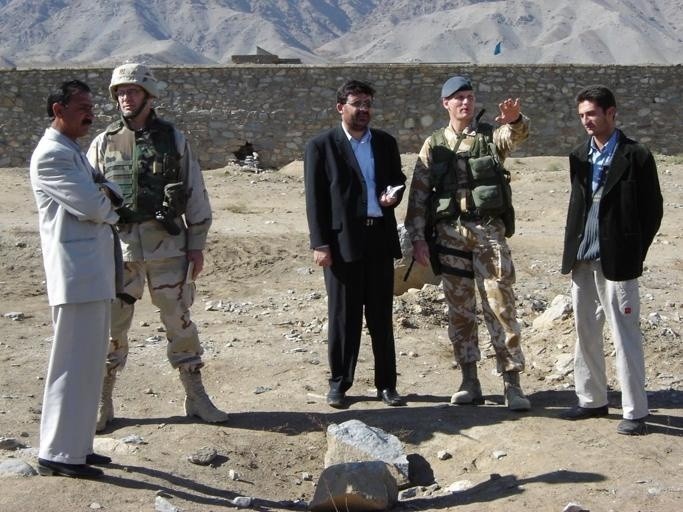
[
  {"xmin": 29, "ymin": 77, "xmax": 124, "ymax": 478},
  {"xmin": 305, "ymin": 80, "xmax": 407, "ymax": 407},
  {"xmin": 404, "ymin": 76, "xmax": 532, "ymax": 409},
  {"xmin": 562, "ymin": 84, "xmax": 663, "ymax": 436},
  {"xmin": 87, "ymin": 63, "xmax": 228, "ymax": 433}
]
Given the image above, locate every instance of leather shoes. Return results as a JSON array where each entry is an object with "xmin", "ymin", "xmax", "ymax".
[
  {"xmin": 377, "ymin": 388, "xmax": 401, "ymax": 406},
  {"xmin": 38, "ymin": 458, "xmax": 105, "ymax": 479},
  {"xmin": 617, "ymin": 418, "xmax": 646, "ymax": 434},
  {"xmin": 85, "ymin": 452, "xmax": 113, "ymax": 465},
  {"xmin": 559, "ymin": 404, "xmax": 608, "ymax": 420},
  {"xmin": 327, "ymin": 389, "xmax": 345, "ymax": 407}
]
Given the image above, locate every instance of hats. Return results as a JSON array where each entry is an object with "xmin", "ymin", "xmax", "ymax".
[{"xmin": 441, "ymin": 76, "xmax": 473, "ymax": 99}]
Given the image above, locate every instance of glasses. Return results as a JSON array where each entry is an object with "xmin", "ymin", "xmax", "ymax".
[{"xmin": 114, "ymin": 89, "xmax": 142, "ymax": 96}]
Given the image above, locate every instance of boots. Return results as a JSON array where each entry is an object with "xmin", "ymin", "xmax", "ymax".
[
  {"xmin": 503, "ymin": 370, "xmax": 531, "ymax": 410},
  {"xmin": 181, "ymin": 372, "xmax": 229, "ymax": 424},
  {"xmin": 450, "ymin": 363, "xmax": 483, "ymax": 404},
  {"xmin": 96, "ymin": 376, "xmax": 116, "ymax": 432}
]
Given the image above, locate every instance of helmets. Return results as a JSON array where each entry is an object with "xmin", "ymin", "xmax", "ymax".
[{"xmin": 109, "ymin": 63, "xmax": 159, "ymax": 101}]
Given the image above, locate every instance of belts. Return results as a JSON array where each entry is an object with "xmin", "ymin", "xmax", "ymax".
[{"xmin": 364, "ymin": 216, "xmax": 382, "ymax": 228}]
